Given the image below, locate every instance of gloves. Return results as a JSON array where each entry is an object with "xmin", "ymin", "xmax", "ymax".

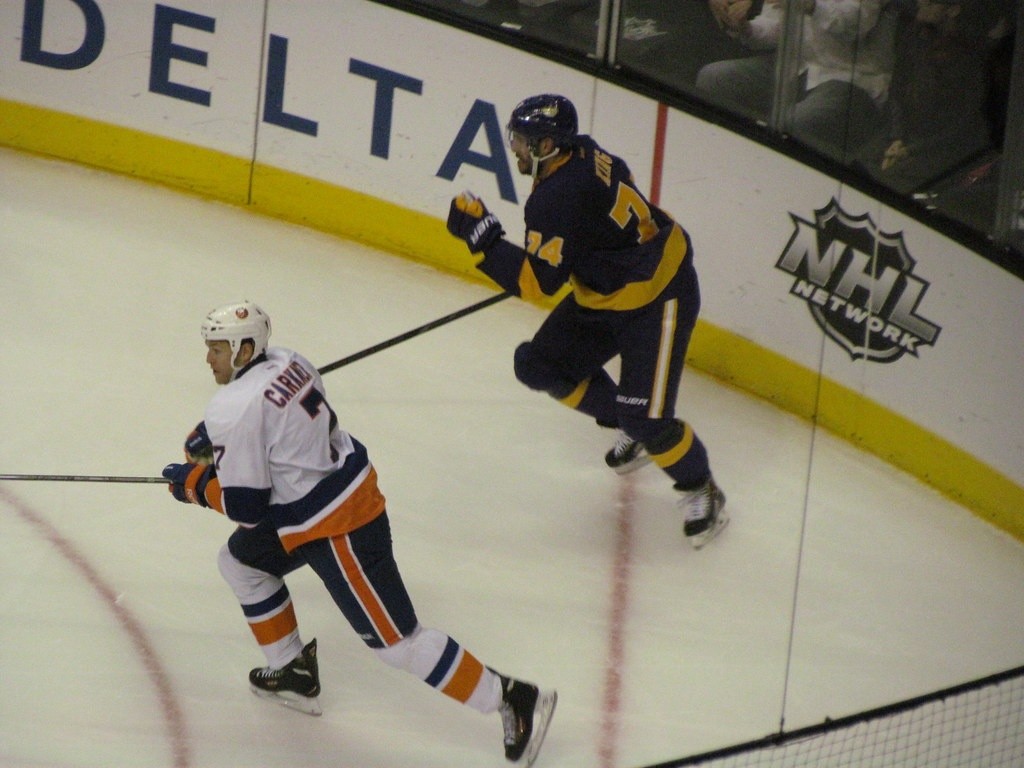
[
  {"xmin": 184, "ymin": 420, "xmax": 214, "ymax": 467},
  {"xmin": 447, "ymin": 190, "xmax": 506, "ymax": 254},
  {"xmin": 162, "ymin": 463, "xmax": 217, "ymax": 509}
]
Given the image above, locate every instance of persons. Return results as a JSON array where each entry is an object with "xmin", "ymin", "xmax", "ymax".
[
  {"xmin": 162, "ymin": 302, "xmax": 559, "ymax": 768},
  {"xmin": 446, "ymin": 94, "xmax": 731, "ymax": 549},
  {"xmin": 429, "ymin": 1, "xmax": 1024, "ymax": 260}
]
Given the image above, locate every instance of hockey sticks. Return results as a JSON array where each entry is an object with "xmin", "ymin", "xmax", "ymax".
[
  {"xmin": 317, "ymin": 290, "xmax": 514, "ymax": 377},
  {"xmin": 0, "ymin": 473, "xmax": 174, "ymax": 485}
]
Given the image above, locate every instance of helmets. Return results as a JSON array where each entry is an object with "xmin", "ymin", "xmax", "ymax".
[
  {"xmin": 505, "ymin": 94, "xmax": 578, "ymax": 153},
  {"xmin": 201, "ymin": 300, "xmax": 272, "ymax": 383}
]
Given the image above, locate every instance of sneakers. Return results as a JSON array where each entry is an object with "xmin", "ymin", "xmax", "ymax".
[
  {"xmin": 673, "ymin": 471, "xmax": 730, "ymax": 549},
  {"xmin": 249, "ymin": 638, "xmax": 323, "ymax": 716},
  {"xmin": 605, "ymin": 431, "xmax": 653, "ymax": 474},
  {"xmin": 486, "ymin": 667, "xmax": 558, "ymax": 768}
]
